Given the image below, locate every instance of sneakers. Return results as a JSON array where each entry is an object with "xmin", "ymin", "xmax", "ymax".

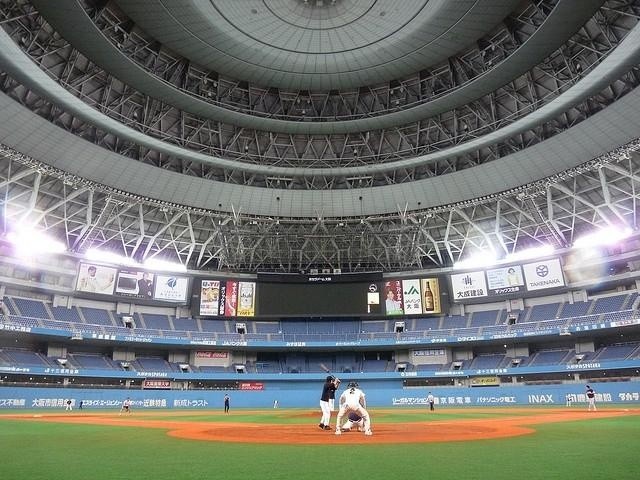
[{"xmin": 319, "ymin": 424, "xmax": 372, "ymax": 436}]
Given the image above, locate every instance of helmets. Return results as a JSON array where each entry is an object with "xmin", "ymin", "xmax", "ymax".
[{"xmin": 348, "ymin": 383, "xmax": 359, "ymax": 388}]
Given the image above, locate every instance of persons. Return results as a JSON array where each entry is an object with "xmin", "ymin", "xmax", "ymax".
[
  {"xmin": 117, "ymin": 397, "xmax": 132, "ymax": 416},
  {"xmin": 78, "ymin": 401, "xmax": 83, "ymax": 410},
  {"xmin": 78, "ymin": 266, "xmax": 114, "ymax": 293},
  {"xmin": 585, "ymin": 385, "xmax": 596, "ymax": 411},
  {"xmin": 137, "ymin": 272, "xmax": 152, "ymax": 297},
  {"xmin": 506, "ymin": 268, "xmax": 520, "ymax": 286},
  {"xmin": 342, "ymin": 411, "xmax": 365, "ymax": 432},
  {"xmin": 317, "ymin": 375, "xmax": 339, "ymax": 431},
  {"xmin": 426, "ymin": 392, "xmax": 434, "ymax": 412},
  {"xmin": 333, "ymin": 381, "xmax": 373, "ymax": 436},
  {"xmin": 385, "ymin": 289, "xmax": 401, "ymax": 311},
  {"xmin": 223, "ymin": 393, "xmax": 230, "ymax": 414},
  {"xmin": 66, "ymin": 399, "xmax": 72, "ymax": 411}
]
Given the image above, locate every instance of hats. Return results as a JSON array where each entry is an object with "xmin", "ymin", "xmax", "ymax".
[{"xmin": 327, "ymin": 376, "xmax": 333, "ymax": 382}]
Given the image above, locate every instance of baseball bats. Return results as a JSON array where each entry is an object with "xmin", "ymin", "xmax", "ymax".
[{"xmin": 319, "ymin": 364, "xmax": 340, "ymax": 383}]
